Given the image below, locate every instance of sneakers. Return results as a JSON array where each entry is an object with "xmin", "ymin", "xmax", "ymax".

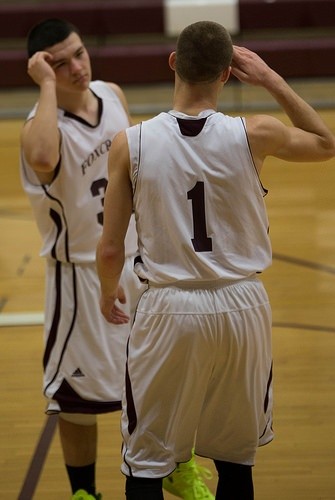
[
  {"xmin": 163, "ymin": 449, "xmax": 215, "ymax": 500},
  {"xmin": 71, "ymin": 490, "xmax": 102, "ymax": 500}
]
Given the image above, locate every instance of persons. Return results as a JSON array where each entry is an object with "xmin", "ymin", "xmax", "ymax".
[
  {"xmin": 20, "ymin": 18, "xmax": 215, "ymax": 500},
  {"xmin": 96, "ymin": 21, "xmax": 334, "ymax": 500}
]
